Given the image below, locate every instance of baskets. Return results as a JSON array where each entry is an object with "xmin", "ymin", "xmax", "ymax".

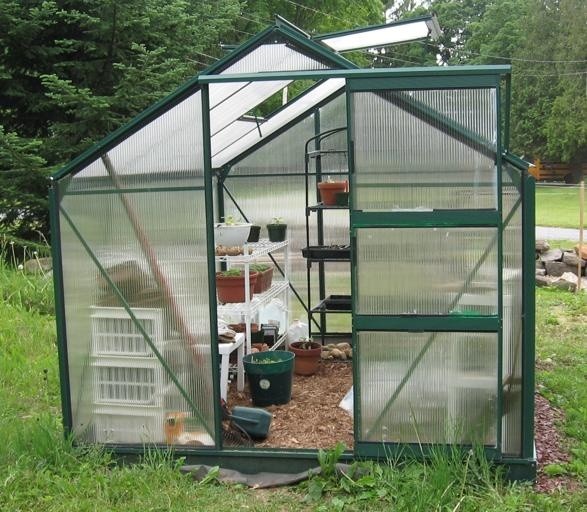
[{"xmin": 89, "ymin": 304, "xmax": 188, "ymax": 445}]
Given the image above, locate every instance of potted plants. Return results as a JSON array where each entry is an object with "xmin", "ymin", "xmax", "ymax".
[
  {"xmin": 215, "ymin": 263, "xmax": 273, "ymax": 303},
  {"xmin": 299, "ymin": 240, "xmax": 350, "ymax": 259},
  {"xmin": 214, "ymin": 214, "xmax": 287, "ymax": 249},
  {"xmin": 317, "ymin": 175, "xmax": 346, "ymax": 206},
  {"xmin": 289, "ymin": 334, "xmax": 322, "ymax": 376}
]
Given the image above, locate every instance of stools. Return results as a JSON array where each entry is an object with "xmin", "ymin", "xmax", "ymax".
[{"xmin": 188, "ymin": 332, "xmax": 246, "ymax": 405}]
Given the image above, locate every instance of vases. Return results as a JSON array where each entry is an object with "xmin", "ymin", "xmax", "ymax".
[
  {"xmin": 325, "ymin": 295, "xmax": 352, "ymax": 310},
  {"xmin": 242, "ymin": 349, "xmax": 296, "ymax": 407},
  {"xmin": 335, "ymin": 192, "xmax": 349, "ymax": 206}
]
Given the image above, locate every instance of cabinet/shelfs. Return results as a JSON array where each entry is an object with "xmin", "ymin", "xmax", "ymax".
[
  {"xmin": 304, "ymin": 127, "xmax": 352, "ymax": 347},
  {"xmin": 213, "ymin": 229, "xmax": 289, "ymax": 359},
  {"xmin": 444, "ymin": 292, "xmax": 513, "ymax": 453}
]
